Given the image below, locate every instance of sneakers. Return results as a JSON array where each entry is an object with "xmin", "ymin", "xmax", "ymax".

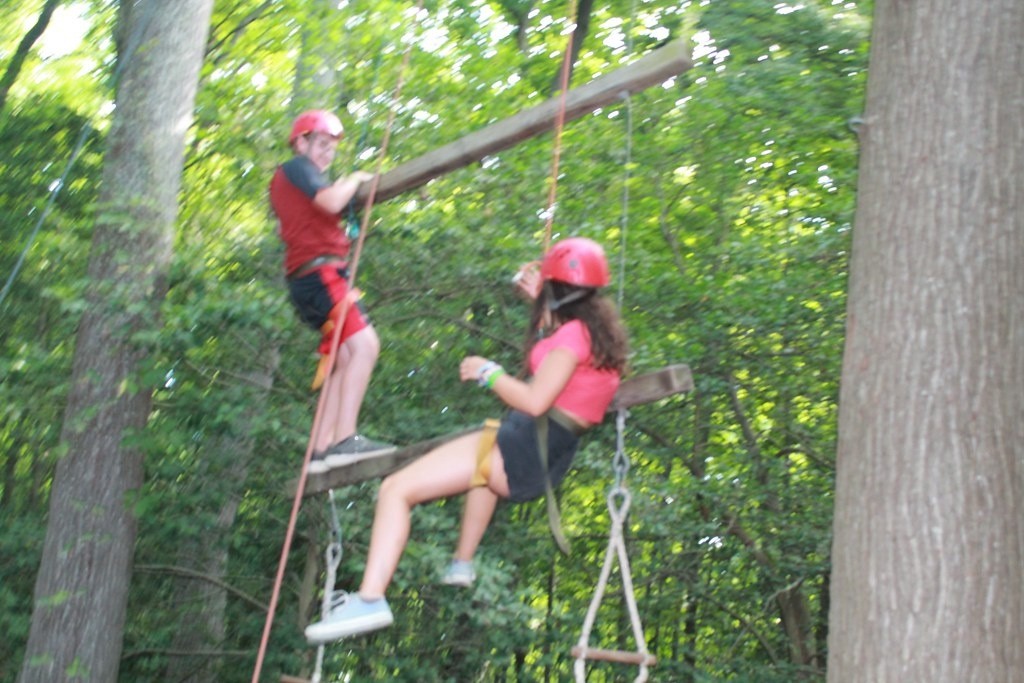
[
  {"xmin": 427, "ymin": 561, "xmax": 478, "ymax": 593},
  {"xmin": 307, "ymin": 432, "xmax": 398, "ymax": 474},
  {"xmin": 304, "ymin": 589, "xmax": 393, "ymax": 640}
]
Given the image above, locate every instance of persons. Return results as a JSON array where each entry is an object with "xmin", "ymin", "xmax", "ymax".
[
  {"xmin": 305, "ymin": 240, "xmax": 625, "ymax": 640},
  {"xmin": 270, "ymin": 110, "xmax": 398, "ymax": 473}
]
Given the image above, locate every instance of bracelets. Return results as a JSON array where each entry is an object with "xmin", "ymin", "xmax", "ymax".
[{"xmin": 478, "ymin": 361, "xmax": 504, "ymax": 390}]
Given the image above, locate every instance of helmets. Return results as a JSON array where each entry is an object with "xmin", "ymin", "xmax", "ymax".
[
  {"xmin": 540, "ymin": 236, "xmax": 609, "ymax": 288},
  {"xmin": 288, "ymin": 109, "xmax": 344, "ymax": 145}
]
